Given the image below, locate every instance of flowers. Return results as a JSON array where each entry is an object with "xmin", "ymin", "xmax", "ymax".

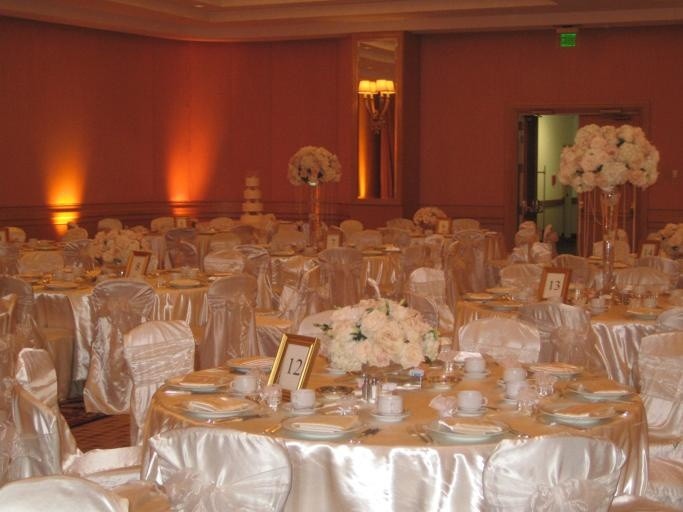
[
  {"xmin": 559, "ymin": 124, "xmax": 660, "ymax": 194},
  {"xmin": 319, "ymin": 296, "xmax": 440, "ymax": 375},
  {"xmin": 287, "ymin": 144, "xmax": 342, "ymax": 188}
]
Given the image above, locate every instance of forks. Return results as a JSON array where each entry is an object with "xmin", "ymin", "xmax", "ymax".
[
  {"xmin": 534, "ymin": 414, "xmax": 586, "ymax": 431},
  {"xmin": 404, "ymin": 423, "xmax": 434, "ymax": 444}
]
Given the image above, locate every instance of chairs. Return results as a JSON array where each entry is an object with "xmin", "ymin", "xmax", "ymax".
[
  {"xmin": 1, "ymin": 474, "xmax": 126, "ymax": 512},
  {"xmin": 14, "ymin": 348, "xmax": 141, "ymax": 472},
  {"xmin": 139, "ymin": 427, "xmax": 292, "ymax": 512},
  {"xmin": 0, "ymin": 215, "xmax": 680, "ymax": 365},
  {"xmin": 634, "ymin": 332, "xmax": 682, "ymax": 510},
  {"xmin": 3, "ymin": 272, "xmax": 72, "ymax": 406},
  {"xmin": 83, "ymin": 278, "xmax": 155, "ymax": 416},
  {"xmin": 124, "ymin": 319, "xmax": 196, "ymax": 442},
  {"xmin": 483, "ymin": 437, "xmax": 624, "ymax": 512},
  {"xmin": 1, "ymin": 379, "xmax": 146, "ymax": 484}
]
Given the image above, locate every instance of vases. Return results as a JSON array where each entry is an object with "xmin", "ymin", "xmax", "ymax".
[
  {"xmin": 309, "ymin": 187, "xmax": 321, "ymax": 245},
  {"xmin": 599, "ymin": 194, "xmax": 619, "ymax": 291}
]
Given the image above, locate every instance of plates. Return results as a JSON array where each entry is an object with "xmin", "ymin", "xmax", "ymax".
[
  {"xmin": 224, "ymin": 354, "xmax": 277, "ymax": 373},
  {"xmin": 459, "ymin": 369, "xmax": 492, "ymax": 380},
  {"xmin": 453, "ymin": 408, "xmax": 487, "ymax": 417},
  {"xmin": 316, "ymin": 386, "xmax": 353, "ymax": 400},
  {"xmin": 5, "ymin": 265, "xmax": 232, "ymax": 290},
  {"xmin": 422, "ymin": 416, "xmax": 511, "ymax": 442},
  {"xmin": 282, "ymin": 415, "xmax": 365, "ymax": 438},
  {"xmin": 369, "ymin": 407, "xmax": 411, "ymax": 424},
  {"xmin": 462, "ymin": 286, "xmax": 667, "ymax": 319},
  {"xmin": 286, "ymin": 403, "xmax": 316, "ymax": 415},
  {"xmin": 163, "ymin": 375, "xmax": 232, "ymax": 393},
  {"xmin": 529, "ymin": 362, "xmax": 583, "ymax": 377},
  {"xmin": 537, "ymin": 398, "xmax": 617, "ymax": 426},
  {"xmin": 564, "ymin": 380, "xmax": 634, "ymax": 402},
  {"xmin": 180, "ymin": 395, "xmax": 258, "ymax": 420}
]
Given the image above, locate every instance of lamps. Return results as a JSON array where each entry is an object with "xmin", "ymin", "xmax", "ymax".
[
  {"xmin": 357, "ymin": 78, "xmax": 389, "ymax": 119},
  {"xmin": 368, "ymin": 81, "xmax": 395, "ymax": 118}
]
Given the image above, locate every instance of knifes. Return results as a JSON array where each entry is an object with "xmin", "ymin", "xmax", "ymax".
[
  {"xmin": 205, "ymin": 414, "xmax": 261, "ymax": 425},
  {"xmin": 348, "ymin": 428, "xmax": 378, "ymax": 444},
  {"xmin": 263, "ymin": 417, "xmax": 294, "ymax": 434}
]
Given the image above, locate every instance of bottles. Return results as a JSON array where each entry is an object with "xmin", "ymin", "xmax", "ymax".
[{"xmin": 361, "ymin": 376, "xmax": 382, "ymax": 405}]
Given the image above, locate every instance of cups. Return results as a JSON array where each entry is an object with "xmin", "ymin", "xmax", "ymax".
[
  {"xmin": 376, "ymin": 393, "xmax": 403, "ymax": 416},
  {"xmin": 455, "ymin": 389, "xmax": 489, "ymax": 412},
  {"xmin": 289, "ymin": 388, "xmax": 316, "ymax": 409},
  {"xmin": 499, "ymin": 366, "xmax": 558, "ymax": 414},
  {"xmin": 426, "ymin": 357, "xmax": 460, "ymax": 390},
  {"xmin": 463, "ymin": 356, "xmax": 487, "ymax": 373},
  {"xmin": 227, "ymin": 367, "xmax": 282, "ymax": 413}
]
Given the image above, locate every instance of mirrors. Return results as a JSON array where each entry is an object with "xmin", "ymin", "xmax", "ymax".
[{"xmin": 358, "ymin": 43, "xmax": 396, "ymax": 200}]
